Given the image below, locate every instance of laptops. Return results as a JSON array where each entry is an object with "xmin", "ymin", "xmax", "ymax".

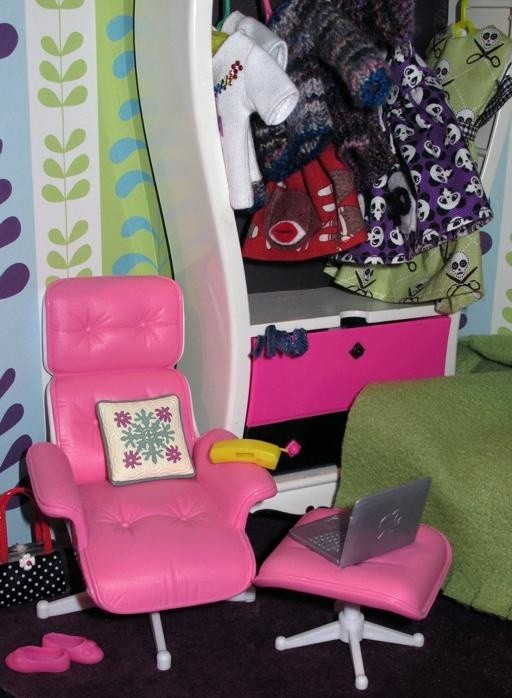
[{"xmin": 289, "ymin": 476, "xmax": 432, "ymax": 568}]
